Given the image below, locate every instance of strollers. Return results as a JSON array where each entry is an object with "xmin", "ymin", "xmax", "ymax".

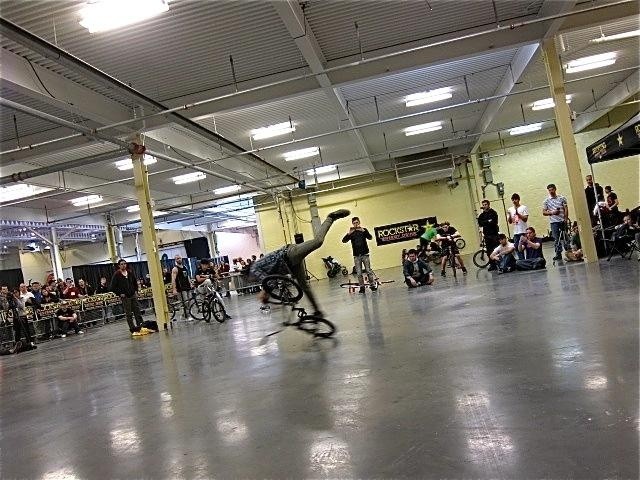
[{"xmin": 322, "ymin": 256, "xmax": 348, "ymax": 278}]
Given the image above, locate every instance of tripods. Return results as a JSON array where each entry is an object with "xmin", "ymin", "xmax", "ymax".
[{"xmin": 0, "ymin": 303, "xmax": 33, "ymax": 349}]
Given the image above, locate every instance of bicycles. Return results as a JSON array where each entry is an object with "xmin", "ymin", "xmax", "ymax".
[
  {"xmin": 432, "ymin": 236, "xmax": 465, "ymax": 264},
  {"xmin": 153, "ymin": 293, "xmax": 208, "ymax": 321},
  {"xmin": 473, "ymin": 232, "xmax": 490, "ymax": 268},
  {"xmin": 340, "ymin": 276, "xmax": 395, "ymax": 293},
  {"xmin": 190, "ymin": 278, "xmax": 226, "ymax": 322},
  {"xmin": 259, "ymin": 274, "xmax": 335, "ymax": 338}
]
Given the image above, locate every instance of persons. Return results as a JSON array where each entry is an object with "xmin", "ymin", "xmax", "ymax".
[
  {"xmin": 477, "ymin": 174, "xmax": 639, "ymax": 274},
  {"xmin": 0, "ymin": 253, "xmax": 266, "ymax": 356},
  {"xmin": 419, "ymin": 223, "xmax": 440, "ymax": 256},
  {"xmin": 342, "ymin": 217, "xmax": 377, "ymax": 294},
  {"xmin": 403, "ymin": 249, "xmax": 434, "ymax": 287},
  {"xmin": 241, "ymin": 208, "xmax": 351, "ymax": 319},
  {"xmin": 436, "ymin": 220, "xmax": 467, "ymax": 277}
]
